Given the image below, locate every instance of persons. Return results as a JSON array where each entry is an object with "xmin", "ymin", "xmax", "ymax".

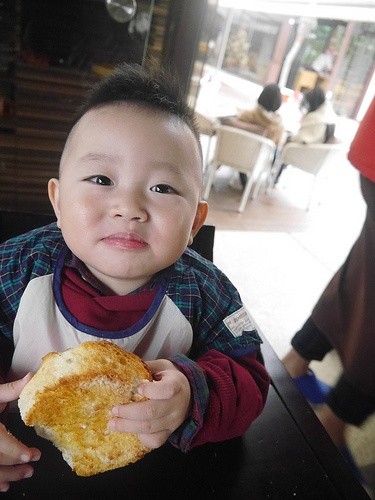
[
  {"xmin": 271, "ymin": 87, "xmax": 336, "ymax": 189},
  {"xmin": 0, "ymin": 74, "xmax": 270, "ymax": 493},
  {"xmin": 280, "ymin": 94, "xmax": 375, "ymax": 484},
  {"xmin": 238, "ymin": 84, "xmax": 284, "ymax": 196}
]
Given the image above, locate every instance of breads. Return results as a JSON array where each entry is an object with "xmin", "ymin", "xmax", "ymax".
[{"xmin": 18, "ymin": 342, "xmax": 155, "ymax": 476}]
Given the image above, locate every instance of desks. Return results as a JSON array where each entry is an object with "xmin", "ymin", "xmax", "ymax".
[{"xmin": 0, "ymin": 330, "xmax": 374, "ymax": 500}]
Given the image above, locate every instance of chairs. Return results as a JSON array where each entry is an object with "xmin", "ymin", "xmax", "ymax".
[{"xmin": 194, "ymin": 109, "xmax": 340, "ymax": 212}]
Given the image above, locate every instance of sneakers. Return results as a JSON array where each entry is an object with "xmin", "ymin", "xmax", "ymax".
[{"xmin": 291, "ymin": 367, "xmax": 333, "ymax": 405}]
